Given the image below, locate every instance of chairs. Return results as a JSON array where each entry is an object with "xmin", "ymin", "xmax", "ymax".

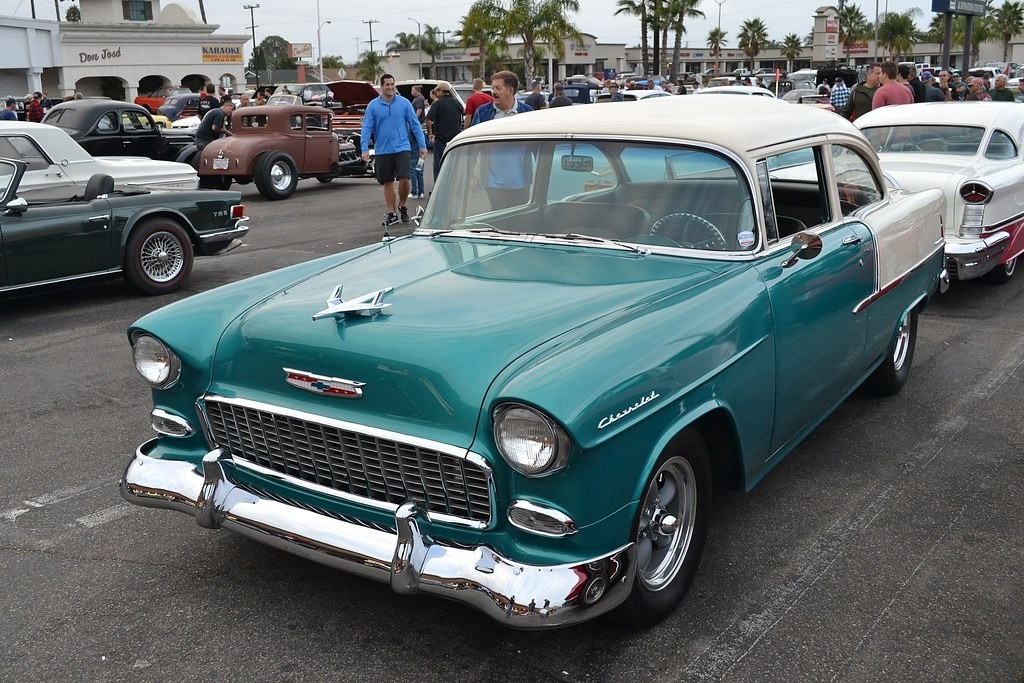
[{"xmin": 245, "ymin": 114, "xmax": 1019, "ymax": 241}]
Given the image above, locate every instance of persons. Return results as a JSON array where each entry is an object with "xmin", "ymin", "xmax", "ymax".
[
  {"xmin": 409, "ymin": 83, "xmax": 426, "ymax": 128},
  {"xmin": 404, "ymin": 120, "xmax": 434, "ymax": 199},
  {"xmin": 359, "ymin": 70, "xmax": 428, "ymax": 226},
  {"xmin": 818, "ymin": 63, "xmax": 1024, "ymax": 149},
  {"xmin": 0, "ymin": 91, "xmax": 83, "ymax": 122},
  {"xmin": 460, "ymin": 70, "xmax": 539, "ymax": 215},
  {"xmin": 424, "ymin": 89, "xmax": 440, "ymax": 108},
  {"xmin": 548, "ymin": 71, "xmax": 789, "ymax": 109},
  {"xmin": 464, "ymin": 77, "xmax": 494, "ymax": 189},
  {"xmin": 195, "ymin": 83, "xmax": 313, "ymax": 153},
  {"xmin": 425, "ymin": 81, "xmax": 464, "ymax": 196},
  {"xmin": 522, "ymin": 80, "xmax": 547, "ymax": 110}
]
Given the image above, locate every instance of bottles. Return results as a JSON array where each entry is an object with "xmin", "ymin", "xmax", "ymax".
[{"xmin": 414, "ymin": 155, "xmax": 424, "ymax": 171}]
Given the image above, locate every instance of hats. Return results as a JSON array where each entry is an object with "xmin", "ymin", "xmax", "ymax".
[
  {"xmin": 607, "ymin": 83, "xmax": 619, "ymax": 88},
  {"xmin": 434, "ymin": 82, "xmax": 451, "ymax": 91},
  {"xmin": 6, "ymin": 98, "xmax": 18, "ymax": 103},
  {"xmin": 34, "ymin": 92, "xmax": 42, "ymax": 99}
]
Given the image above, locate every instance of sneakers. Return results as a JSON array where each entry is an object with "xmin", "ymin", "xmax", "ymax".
[{"xmin": 382, "ymin": 205, "xmax": 409, "ymax": 226}]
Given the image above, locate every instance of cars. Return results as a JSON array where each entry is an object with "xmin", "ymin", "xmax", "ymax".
[
  {"xmin": 0, "ymin": 156, "xmax": 250, "ymax": 297},
  {"xmin": 199, "ymin": 104, "xmax": 385, "ymax": 201},
  {"xmin": 0, "ymin": 119, "xmax": 201, "ymax": 201},
  {"xmin": 0, "ymin": 79, "xmax": 493, "ymax": 185},
  {"xmin": 117, "ymin": 92, "xmax": 951, "ymax": 639},
  {"xmin": 760, "ymin": 102, "xmax": 1024, "ymax": 283},
  {"xmin": 516, "ymin": 60, "xmax": 1024, "ymax": 123}
]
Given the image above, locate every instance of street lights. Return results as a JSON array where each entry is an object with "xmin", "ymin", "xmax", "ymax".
[{"xmin": 318, "ymin": 21, "xmax": 332, "ymax": 84}]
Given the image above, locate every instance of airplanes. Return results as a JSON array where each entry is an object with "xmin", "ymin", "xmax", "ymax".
[{"xmin": 311, "ymin": 282, "xmax": 396, "ymax": 325}]
